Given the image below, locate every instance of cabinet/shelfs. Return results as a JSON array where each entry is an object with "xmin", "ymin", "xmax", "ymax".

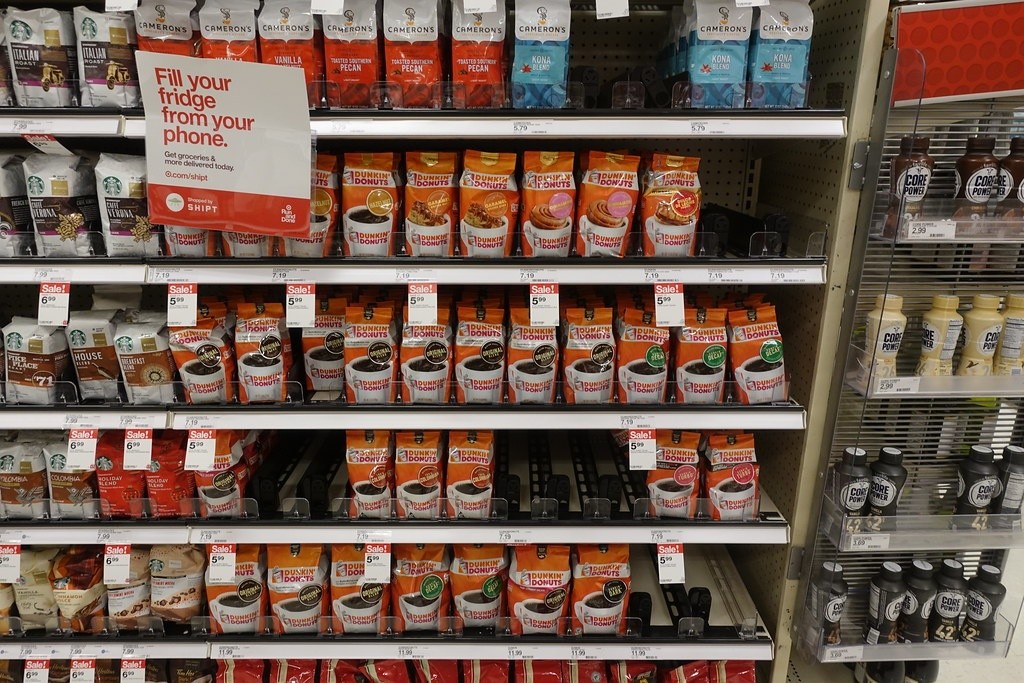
[{"xmin": 0, "ymin": 0, "xmax": 1024, "ymax": 683}]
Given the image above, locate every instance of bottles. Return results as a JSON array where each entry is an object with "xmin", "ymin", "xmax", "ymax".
[
  {"xmin": 897, "ymin": 560, "xmax": 937, "ymax": 645},
  {"xmin": 808, "ymin": 562, "xmax": 848, "ymax": 646},
  {"xmin": 914, "ymin": 295, "xmax": 964, "ymax": 390},
  {"xmin": 956, "ymin": 294, "xmax": 1004, "ymax": 387},
  {"xmin": 993, "ymin": 293, "xmax": 1024, "ymax": 386},
  {"xmin": 856, "ymin": 294, "xmax": 907, "ymax": 388},
  {"xmin": 989, "ymin": 445, "xmax": 1024, "ymax": 533},
  {"xmin": 950, "ymin": 138, "xmax": 1000, "ymax": 236},
  {"xmin": 960, "ymin": 565, "xmax": 1006, "ymax": 654},
  {"xmin": 929, "ymin": 559, "xmax": 966, "ymax": 644},
  {"xmin": 863, "ymin": 446, "xmax": 908, "ymax": 533},
  {"xmin": 990, "ymin": 137, "xmax": 1024, "ymax": 237},
  {"xmin": 883, "ymin": 137, "xmax": 935, "ymax": 239},
  {"xmin": 949, "ymin": 445, "xmax": 998, "ymax": 535},
  {"xmin": 863, "ymin": 562, "xmax": 906, "ymax": 646},
  {"xmin": 825, "ymin": 447, "xmax": 870, "ymax": 545},
  {"xmin": 843, "ymin": 661, "xmax": 939, "ymax": 683}
]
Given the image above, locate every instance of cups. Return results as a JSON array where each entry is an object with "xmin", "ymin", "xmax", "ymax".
[{"xmin": 177, "ymin": 208, "xmax": 784, "ymax": 633}]
